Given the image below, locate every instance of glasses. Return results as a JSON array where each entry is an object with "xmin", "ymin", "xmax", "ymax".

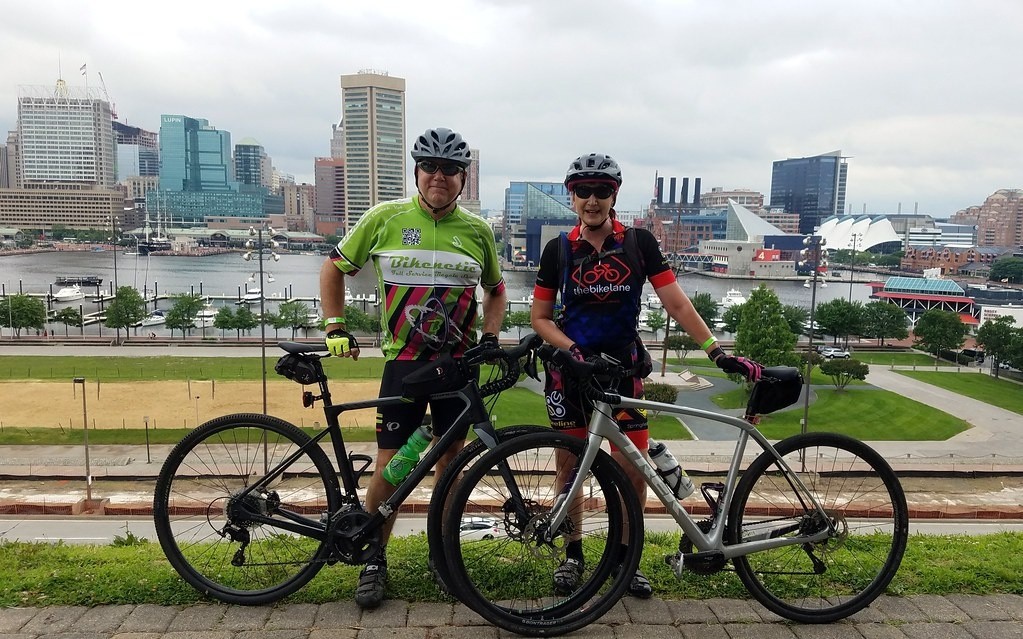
[
  {"xmin": 573, "ymin": 185, "xmax": 615, "ymax": 200},
  {"xmin": 417, "ymin": 161, "xmax": 466, "ymax": 177}
]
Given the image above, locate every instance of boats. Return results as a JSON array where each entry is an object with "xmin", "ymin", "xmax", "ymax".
[
  {"xmin": 710, "ymin": 287, "xmax": 748, "ymax": 332},
  {"xmin": 527, "ymin": 290, "xmax": 534, "ymax": 307},
  {"xmin": 638, "ymin": 310, "xmax": 679, "ymax": 333},
  {"xmin": 178, "ymin": 299, "xmax": 220, "ymax": 330},
  {"xmin": 646, "ymin": 293, "xmax": 664, "ymax": 310},
  {"xmin": 131, "ymin": 309, "xmax": 169, "ymax": 328},
  {"xmin": 54, "ymin": 282, "xmax": 86, "ymax": 303},
  {"xmin": 301, "ymin": 307, "xmax": 323, "ymax": 328},
  {"xmin": 53, "ymin": 274, "xmax": 104, "ymax": 287}
]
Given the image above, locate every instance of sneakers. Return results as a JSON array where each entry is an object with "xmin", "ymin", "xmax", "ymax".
[
  {"xmin": 611, "ymin": 564, "xmax": 652, "ymax": 599},
  {"xmin": 355, "ymin": 565, "xmax": 388, "ymax": 608},
  {"xmin": 553, "ymin": 558, "xmax": 584, "ymax": 589},
  {"xmin": 427, "ymin": 551, "xmax": 448, "ymax": 592}
]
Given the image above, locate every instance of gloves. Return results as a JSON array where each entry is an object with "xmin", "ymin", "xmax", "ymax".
[
  {"xmin": 715, "ymin": 353, "xmax": 765, "ymax": 383},
  {"xmin": 569, "ymin": 343, "xmax": 583, "ymax": 362}
]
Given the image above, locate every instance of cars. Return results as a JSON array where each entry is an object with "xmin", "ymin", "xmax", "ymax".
[{"xmin": 822, "ymin": 348, "xmax": 851, "ymax": 359}]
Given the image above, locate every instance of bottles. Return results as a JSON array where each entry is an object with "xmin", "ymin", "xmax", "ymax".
[
  {"xmin": 647, "ymin": 437, "xmax": 695, "ymax": 499},
  {"xmin": 381, "ymin": 426, "xmax": 435, "ymax": 487}
]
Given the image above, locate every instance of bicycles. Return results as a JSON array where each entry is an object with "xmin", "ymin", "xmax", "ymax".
[
  {"xmin": 152, "ymin": 337, "xmax": 624, "ymax": 627},
  {"xmin": 442, "ymin": 344, "xmax": 911, "ymax": 639}
]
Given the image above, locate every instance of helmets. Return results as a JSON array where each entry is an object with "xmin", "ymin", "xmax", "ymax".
[
  {"xmin": 564, "ymin": 153, "xmax": 622, "ymax": 191},
  {"xmin": 411, "ymin": 127, "xmax": 472, "ymax": 167}
]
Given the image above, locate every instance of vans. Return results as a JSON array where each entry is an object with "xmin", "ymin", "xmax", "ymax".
[{"xmin": 961, "ymin": 349, "xmax": 984, "ymax": 363}]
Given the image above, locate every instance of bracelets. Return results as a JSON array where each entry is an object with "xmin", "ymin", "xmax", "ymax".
[
  {"xmin": 702, "ymin": 336, "xmax": 718, "ymax": 350},
  {"xmin": 325, "ymin": 317, "xmax": 346, "ymax": 327}
]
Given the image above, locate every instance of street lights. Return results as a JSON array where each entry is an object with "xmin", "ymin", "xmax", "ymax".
[
  {"xmin": 794, "ymin": 232, "xmax": 829, "ymax": 472},
  {"xmin": 848, "ymin": 233, "xmax": 858, "ymax": 303},
  {"xmin": 72, "ymin": 376, "xmax": 96, "ymax": 515},
  {"xmin": 243, "ymin": 222, "xmax": 280, "ymax": 416},
  {"xmin": 106, "ymin": 215, "xmax": 125, "ymax": 346}
]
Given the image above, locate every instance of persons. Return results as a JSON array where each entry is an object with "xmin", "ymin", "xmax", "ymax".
[
  {"xmin": 530, "ymin": 152, "xmax": 765, "ymax": 597},
  {"xmin": 320, "ymin": 128, "xmax": 507, "ymax": 608}
]
